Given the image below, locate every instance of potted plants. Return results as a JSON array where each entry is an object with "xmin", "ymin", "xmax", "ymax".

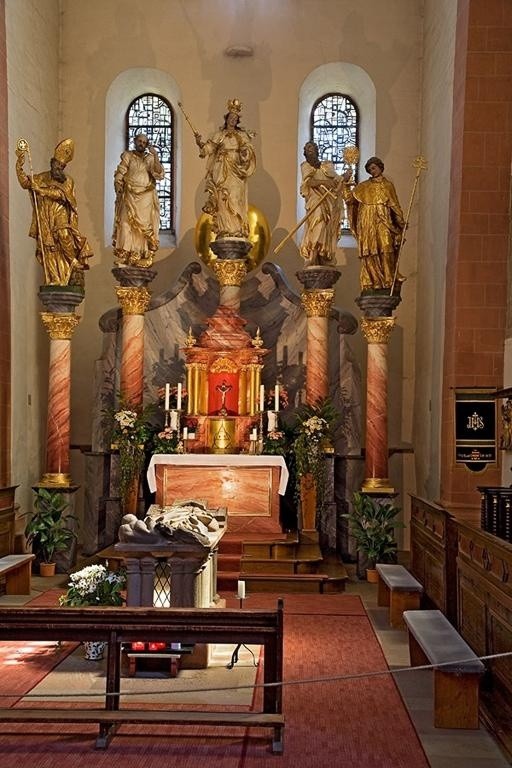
[
  {"xmin": 14, "ymin": 488, "xmax": 81, "ymax": 578},
  {"xmin": 340, "ymin": 490, "xmax": 407, "ymax": 584}
]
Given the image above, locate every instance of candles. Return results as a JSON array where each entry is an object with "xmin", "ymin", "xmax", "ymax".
[
  {"xmin": 165, "ymin": 382, "xmax": 182, "ymax": 411},
  {"xmin": 183, "ymin": 426, "xmax": 188, "ymax": 439},
  {"xmin": 259, "ymin": 384, "xmax": 280, "ymax": 413},
  {"xmin": 237, "ymin": 580, "xmax": 246, "ymax": 598},
  {"xmin": 250, "ymin": 428, "xmax": 257, "ymax": 441}
]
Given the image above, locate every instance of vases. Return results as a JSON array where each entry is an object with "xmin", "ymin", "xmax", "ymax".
[
  {"xmin": 80, "ymin": 640, "xmax": 108, "ymax": 661},
  {"xmin": 117, "ymin": 451, "xmax": 138, "ymax": 516},
  {"xmin": 299, "ymin": 471, "xmax": 319, "ymax": 545}
]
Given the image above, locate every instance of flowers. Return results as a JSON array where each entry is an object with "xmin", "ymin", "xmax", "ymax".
[
  {"xmin": 264, "ymin": 430, "xmax": 288, "ymax": 456},
  {"xmin": 284, "ymin": 394, "xmax": 343, "ymax": 514},
  {"xmin": 96, "ymin": 391, "xmax": 156, "ymax": 508},
  {"xmin": 56, "ymin": 565, "xmax": 126, "ymax": 608},
  {"xmin": 154, "ymin": 428, "xmax": 182, "ymax": 453}
]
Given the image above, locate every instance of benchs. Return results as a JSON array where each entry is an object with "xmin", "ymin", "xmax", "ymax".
[
  {"xmin": 0, "ymin": 553, "xmax": 36, "ymax": 599},
  {"xmin": 374, "ymin": 563, "xmax": 486, "ymax": 731}
]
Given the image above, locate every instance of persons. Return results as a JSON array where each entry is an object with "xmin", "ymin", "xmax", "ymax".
[
  {"xmin": 300, "ymin": 141, "xmax": 352, "ymax": 267},
  {"xmin": 193, "ymin": 98, "xmax": 256, "ymax": 238},
  {"xmin": 112, "ymin": 134, "xmax": 165, "ymax": 268},
  {"xmin": 342, "ymin": 156, "xmax": 409, "ymax": 293},
  {"xmin": 14, "ymin": 137, "xmax": 94, "ymax": 288}
]
